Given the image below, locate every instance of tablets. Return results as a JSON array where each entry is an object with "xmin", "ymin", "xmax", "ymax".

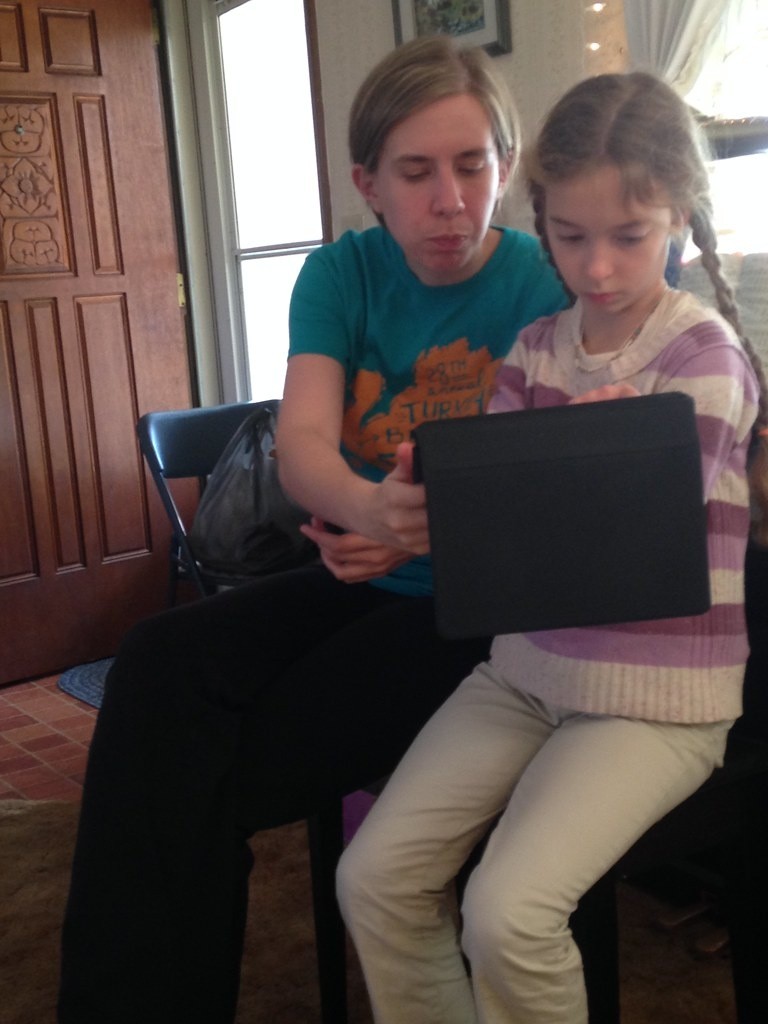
[{"xmin": 410, "ymin": 389, "xmax": 710, "ymax": 643}]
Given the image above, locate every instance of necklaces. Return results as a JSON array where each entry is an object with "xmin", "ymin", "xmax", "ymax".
[{"xmin": 573, "ymin": 285, "xmax": 669, "ymax": 374}]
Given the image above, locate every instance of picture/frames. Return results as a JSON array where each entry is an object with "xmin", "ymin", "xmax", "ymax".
[{"xmin": 391, "ymin": 0, "xmax": 512, "ymax": 58}]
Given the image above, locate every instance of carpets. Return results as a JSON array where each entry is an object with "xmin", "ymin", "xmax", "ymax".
[
  {"xmin": 0, "ymin": 794, "xmax": 747, "ymax": 1024},
  {"xmin": 58, "ymin": 657, "xmax": 114, "ymax": 709}
]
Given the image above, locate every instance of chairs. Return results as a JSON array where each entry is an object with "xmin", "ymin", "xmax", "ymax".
[{"xmin": 135, "ymin": 400, "xmax": 768, "ymax": 1024}]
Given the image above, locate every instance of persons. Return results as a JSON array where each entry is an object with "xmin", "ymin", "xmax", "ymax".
[{"xmin": 58, "ymin": 33, "xmax": 768, "ymax": 1024}]
